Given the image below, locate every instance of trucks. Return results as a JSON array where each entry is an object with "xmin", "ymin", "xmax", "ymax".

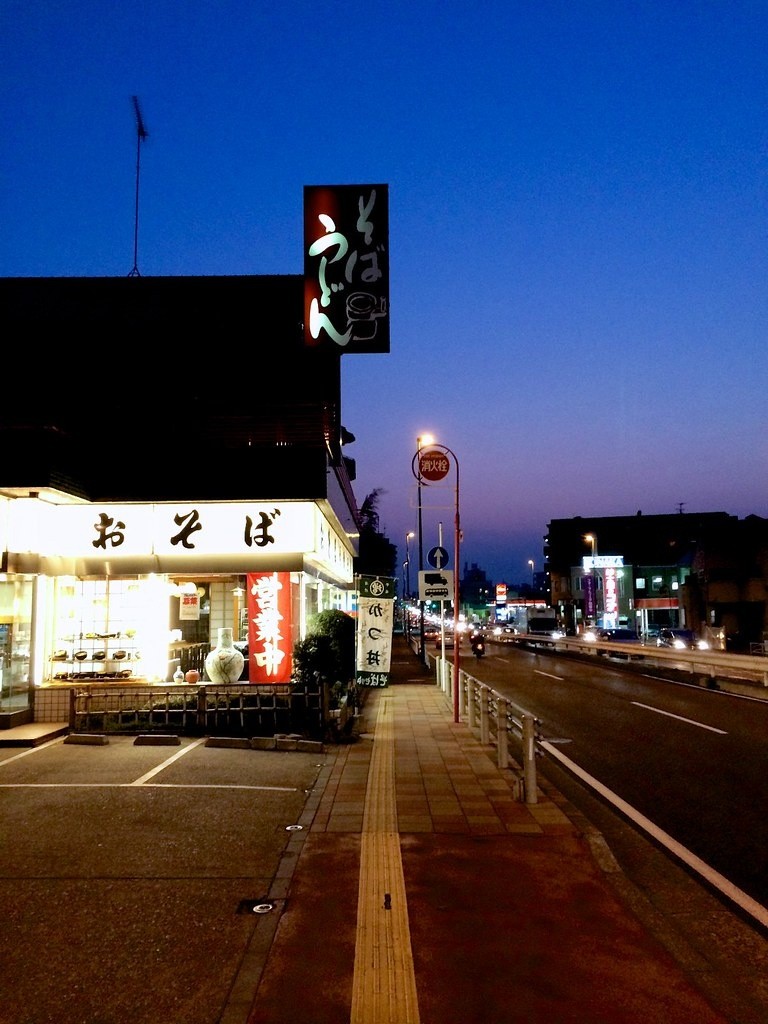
[{"xmin": 517, "ymin": 607, "xmax": 560, "ymax": 645}]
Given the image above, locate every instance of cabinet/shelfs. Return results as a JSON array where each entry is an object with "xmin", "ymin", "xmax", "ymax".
[{"xmin": 1, "ymin": 580, "xmax": 139, "ymax": 692}]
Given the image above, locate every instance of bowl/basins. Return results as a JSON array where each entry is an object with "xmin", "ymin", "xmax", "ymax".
[
  {"xmin": 75, "ymin": 651, "xmax": 87, "ymax": 659},
  {"xmin": 55, "ymin": 649, "xmax": 66, "ymax": 657},
  {"xmin": 56, "ymin": 670, "xmax": 133, "ymax": 678},
  {"xmin": 115, "ymin": 650, "xmax": 126, "ymax": 658},
  {"xmin": 96, "ymin": 651, "xmax": 106, "ymax": 660}
]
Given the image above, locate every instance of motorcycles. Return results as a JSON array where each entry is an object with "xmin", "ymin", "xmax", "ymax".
[{"xmin": 469, "ymin": 633, "xmax": 487, "ymax": 659}]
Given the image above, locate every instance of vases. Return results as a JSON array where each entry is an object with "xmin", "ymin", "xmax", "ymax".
[
  {"xmin": 173, "ymin": 666, "xmax": 184, "ymax": 684},
  {"xmin": 205, "ymin": 627, "xmax": 245, "ymax": 684}
]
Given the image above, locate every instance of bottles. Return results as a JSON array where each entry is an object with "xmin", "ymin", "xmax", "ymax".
[
  {"xmin": 173, "ymin": 666, "xmax": 184, "ymax": 683},
  {"xmin": 206, "ymin": 627, "xmax": 245, "ymax": 684},
  {"xmin": 186, "ymin": 670, "xmax": 199, "ymax": 683}
]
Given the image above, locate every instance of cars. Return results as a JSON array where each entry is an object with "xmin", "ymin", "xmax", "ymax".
[
  {"xmin": 409, "ymin": 611, "xmax": 516, "ymax": 650},
  {"xmin": 644, "ymin": 624, "xmax": 660, "ymax": 637},
  {"xmin": 656, "ymin": 628, "xmax": 712, "ymax": 653},
  {"xmin": 595, "ymin": 627, "xmax": 646, "ymax": 661}
]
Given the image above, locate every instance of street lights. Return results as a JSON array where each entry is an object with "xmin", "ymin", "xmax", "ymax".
[
  {"xmin": 528, "ymin": 559, "xmax": 535, "ymax": 607},
  {"xmin": 416, "ymin": 434, "xmax": 434, "ymax": 665},
  {"xmin": 402, "ymin": 561, "xmax": 407, "ymax": 635},
  {"xmin": 586, "ymin": 534, "xmax": 598, "ymax": 626},
  {"xmin": 406, "ymin": 531, "xmax": 414, "ymax": 646}
]
